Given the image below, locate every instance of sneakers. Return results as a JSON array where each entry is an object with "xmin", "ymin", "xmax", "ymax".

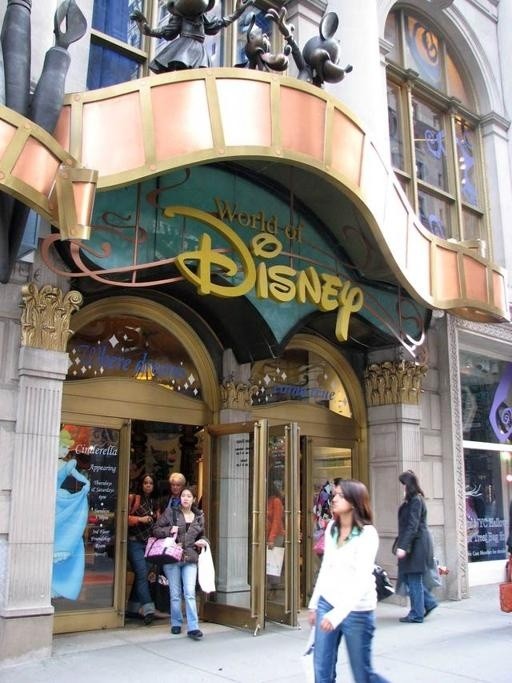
[
  {"xmin": 129, "ymin": 612, "xmax": 153, "ymax": 624},
  {"xmin": 400, "ymin": 605, "xmax": 436, "ymax": 623},
  {"xmin": 171, "ymin": 626, "xmax": 181, "ymax": 634},
  {"xmin": 188, "ymin": 630, "xmax": 203, "ymax": 637}
]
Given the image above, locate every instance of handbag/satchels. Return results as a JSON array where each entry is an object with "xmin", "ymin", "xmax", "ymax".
[
  {"xmin": 398, "ymin": 557, "xmax": 440, "ymax": 595},
  {"xmin": 266, "ymin": 544, "xmax": 285, "ymax": 577},
  {"xmin": 500, "ymin": 583, "xmax": 512, "ymax": 613},
  {"xmin": 198, "ymin": 551, "xmax": 216, "ymax": 594},
  {"xmin": 392, "ymin": 537, "xmax": 411, "ymax": 555},
  {"xmin": 374, "ymin": 568, "xmax": 394, "ymax": 601},
  {"xmin": 144, "ymin": 526, "xmax": 183, "ymax": 564}
]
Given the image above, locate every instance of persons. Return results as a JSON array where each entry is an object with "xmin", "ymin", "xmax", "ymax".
[
  {"xmin": 267, "ymin": 477, "xmax": 286, "ymax": 601},
  {"xmin": 393, "ymin": 470, "xmax": 438, "ymax": 623},
  {"xmin": 51, "ymin": 429, "xmax": 91, "ymax": 601},
  {"xmin": 152, "ymin": 487, "xmax": 210, "ymax": 638},
  {"xmin": 127, "ymin": 473, "xmax": 161, "ymax": 626},
  {"xmin": 154, "ymin": 472, "xmax": 187, "ymax": 614},
  {"xmin": 312, "ymin": 478, "xmax": 343, "ymax": 562},
  {"xmin": 506, "ymin": 500, "xmax": 512, "ymax": 556},
  {"xmin": 308, "ymin": 479, "xmax": 392, "ymax": 683}
]
